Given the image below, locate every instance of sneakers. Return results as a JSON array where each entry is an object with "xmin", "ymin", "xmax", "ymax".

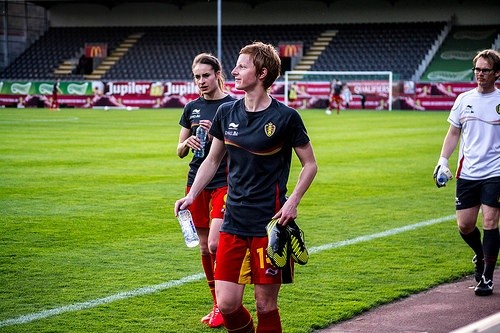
[
  {"xmin": 265, "ymin": 218, "xmax": 289, "ymax": 269},
  {"xmin": 471, "ymin": 254, "xmax": 485, "ymax": 281},
  {"xmin": 286, "ymin": 221, "xmax": 309, "ymax": 265},
  {"xmin": 209, "ymin": 307, "xmax": 225, "ymax": 328},
  {"xmin": 473, "ymin": 275, "xmax": 493, "ymax": 295},
  {"xmin": 201, "ymin": 312, "xmax": 213, "ymax": 323}
]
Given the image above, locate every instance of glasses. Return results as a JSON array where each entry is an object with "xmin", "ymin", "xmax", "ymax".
[{"xmin": 472, "ymin": 66, "xmax": 494, "ymax": 75}]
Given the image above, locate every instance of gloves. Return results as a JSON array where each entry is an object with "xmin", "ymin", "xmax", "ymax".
[{"xmin": 433, "ymin": 157, "xmax": 453, "ymax": 187}]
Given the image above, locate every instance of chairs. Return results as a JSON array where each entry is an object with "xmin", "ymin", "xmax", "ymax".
[{"xmin": 0, "ymin": 20, "xmax": 448, "ymax": 82}]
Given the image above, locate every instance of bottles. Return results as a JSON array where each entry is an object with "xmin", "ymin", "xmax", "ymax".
[
  {"xmin": 176, "ymin": 205, "xmax": 200, "ymax": 248},
  {"xmin": 438, "ymin": 174, "xmax": 448, "ymax": 186},
  {"xmin": 195, "ymin": 126, "xmax": 206, "ymax": 157}
]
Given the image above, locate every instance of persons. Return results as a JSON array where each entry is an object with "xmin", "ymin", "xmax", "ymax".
[
  {"xmin": 360, "ymin": 93, "xmax": 365, "ymax": 109},
  {"xmin": 178, "ymin": 54, "xmax": 237, "ymax": 327},
  {"xmin": 326, "ymin": 79, "xmax": 353, "ymax": 115},
  {"xmin": 50, "ymin": 78, "xmax": 61, "ymax": 110},
  {"xmin": 433, "ymin": 50, "xmax": 500, "ymax": 295},
  {"xmin": 174, "ymin": 42, "xmax": 317, "ymax": 333}
]
